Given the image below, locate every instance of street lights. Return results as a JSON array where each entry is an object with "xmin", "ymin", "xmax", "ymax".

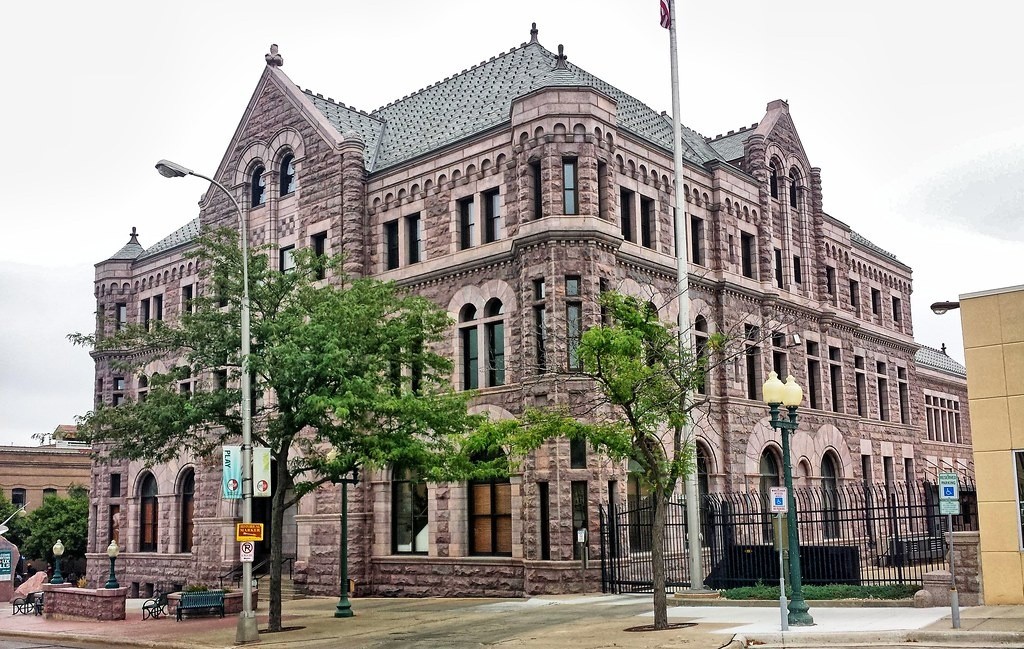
[
  {"xmin": 156, "ymin": 159, "xmax": 261, "ymax": 642},
  {"xmin": 322, "ymin": 443, "xmax": 363, "ymax": 617},
  {"xmin": 762, "ymin": 371, "xmax": 815, "ymax": 628}
]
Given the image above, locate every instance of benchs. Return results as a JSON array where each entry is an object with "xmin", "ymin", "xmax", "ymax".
[
  {"xmin": 177, "ymin": 590, "xmax": 225, "ymax": 622},
  {"xmin": 142, "ymin": 591, "xmax": 174, "ymax": 621},
  {"xmin": 34, "ymin": 593, "xmax": 44, "ymax": 616},
  {"xmin": 13, "ymin": 593, "xmax": 35, "ymax": 614}
]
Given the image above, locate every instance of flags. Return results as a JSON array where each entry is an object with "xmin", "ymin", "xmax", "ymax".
[{"xmin": 660, "ymin": 0, "xmax": 671, "ymax": 29}]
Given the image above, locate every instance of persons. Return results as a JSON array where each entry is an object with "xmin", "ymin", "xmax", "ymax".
[
  {"xmin": 27, "ymin": 562, "xmax": 38, "ymax": 577},
  {"xmin": 14, "ymin": 570, "xmax": 26, "ymax": 587}
]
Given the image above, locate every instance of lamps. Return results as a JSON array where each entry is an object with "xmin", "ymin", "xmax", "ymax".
[
  {"xmin": 51, "ymin": 539, "xmax": 64, "ymax": 584},
  {"xmin": 931, "ymin": 300, "xmax": 959, "ymax": 314},
  {"xmin": 105, "ymin": 540, "xmax": 120, "ymax": 588}
]
